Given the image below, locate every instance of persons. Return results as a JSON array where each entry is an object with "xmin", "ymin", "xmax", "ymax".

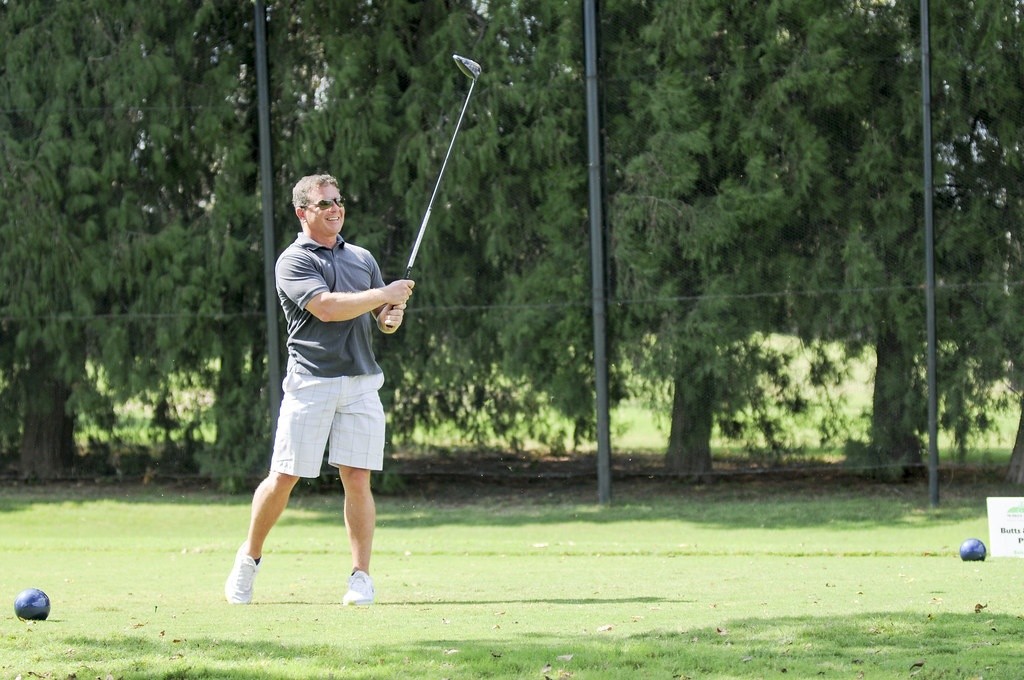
[{"xmin": 224, "ymin": 174, "xmax": 415, "ymax": 608}]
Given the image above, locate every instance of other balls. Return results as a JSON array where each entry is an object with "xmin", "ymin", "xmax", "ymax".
[
  {"xmin": 960, "ymin": 539, "xmax": 986, "ymax": 562},
  {"xmin": 15, "ymin": 588, "xmax": 50, "ymax": 621}
]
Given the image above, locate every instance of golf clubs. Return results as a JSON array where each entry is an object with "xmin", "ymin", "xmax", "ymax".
[{"xmin": 384, "ymin": 54, "xmax": 484, "ymax": 330}]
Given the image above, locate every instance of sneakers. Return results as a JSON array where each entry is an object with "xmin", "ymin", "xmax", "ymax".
[
  {"xmin": 341, "ymin": 569, "xmax": 374, "ymax": 604},
  {"xmin": 224, "ymin": 540, "xmax": 263, "ymax": 605}
]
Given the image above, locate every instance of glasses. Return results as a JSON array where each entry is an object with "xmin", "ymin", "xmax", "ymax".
[{"xmin": 301, "ymin": 196, "xmax": 346, "ymax": 211}]
